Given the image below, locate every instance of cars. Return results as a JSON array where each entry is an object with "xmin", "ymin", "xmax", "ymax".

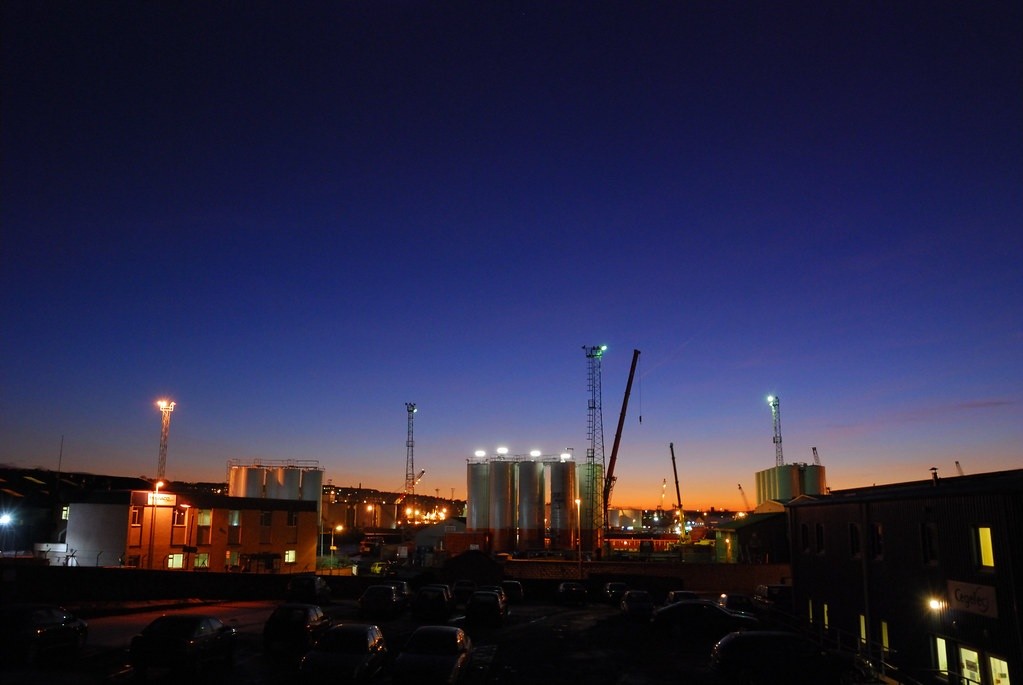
[
  {"xmin": 706, "ymin": 630, "xmax": 876, "ymax": 685},
  {"xmin": 619, "ymin": 591, "xmax": 653, "ymax": 616},
  {"xmin": 559, "ymin": 583, "xmax": 590, "ymax": 600},
  {"xmin": 668, "ymin": 591, "xmax": 699, "ymax": 602},
  {"xmin": 127, "ymin": 573, "xmax": 526, "ymax": 685},
  {"xmin": 602, "ymin": 582, "xmax": 629, "ymax": 602},
  {"xmin": 0, "ymin": 605, "xmax": 89, "ymax": 670},
  {"xmin": 649, "ymin": 599, "xmax": 759, "ymax": 642}
]
